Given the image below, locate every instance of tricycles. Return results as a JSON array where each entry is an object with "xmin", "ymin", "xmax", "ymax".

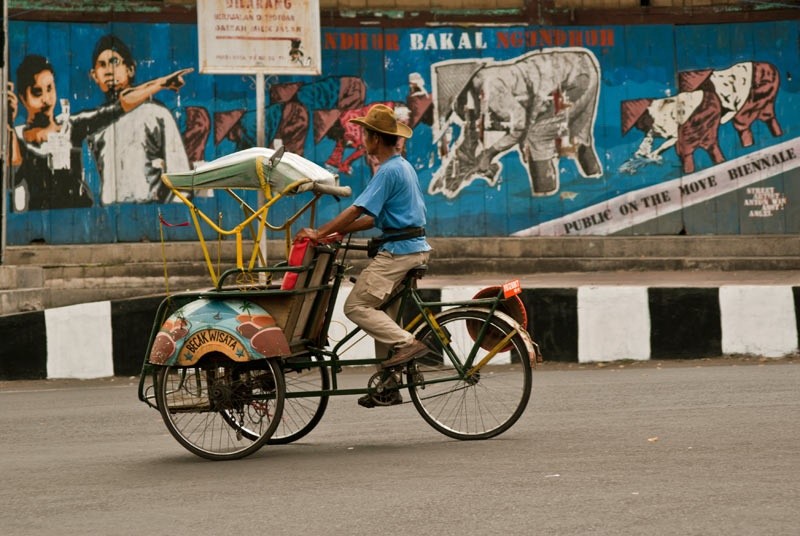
[{"xmin": 137, "ymin": 147, "xmax": 537, "ymax": 461}]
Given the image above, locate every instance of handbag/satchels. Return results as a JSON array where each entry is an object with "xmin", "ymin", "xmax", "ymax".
[{"xmin": 366, "ymin": 228, "xmax": 426, "ymax": 258}]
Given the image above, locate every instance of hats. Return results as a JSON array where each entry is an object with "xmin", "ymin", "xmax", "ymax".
[{"xmin": 350, "ymin": 103, "xmax": 413, "ymax": 138}]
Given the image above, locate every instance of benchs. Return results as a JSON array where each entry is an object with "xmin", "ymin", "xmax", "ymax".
[{"xmin": 280, "ymin": 231, "xmax": 344, "ymax": 290}]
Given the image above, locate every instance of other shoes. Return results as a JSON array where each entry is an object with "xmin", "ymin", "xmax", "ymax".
[
  {"xmin": 380, "ymin": 343, "xmax": 431, "ymax": 368},
  {"xmin": 358, "ymin": 390, "xmax": 402, "ymax": 408}
]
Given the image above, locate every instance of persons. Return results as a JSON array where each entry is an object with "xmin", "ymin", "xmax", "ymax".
[{"xmin": 292, "ymin": 105, "xmax": 432, "ymax": 407}]
